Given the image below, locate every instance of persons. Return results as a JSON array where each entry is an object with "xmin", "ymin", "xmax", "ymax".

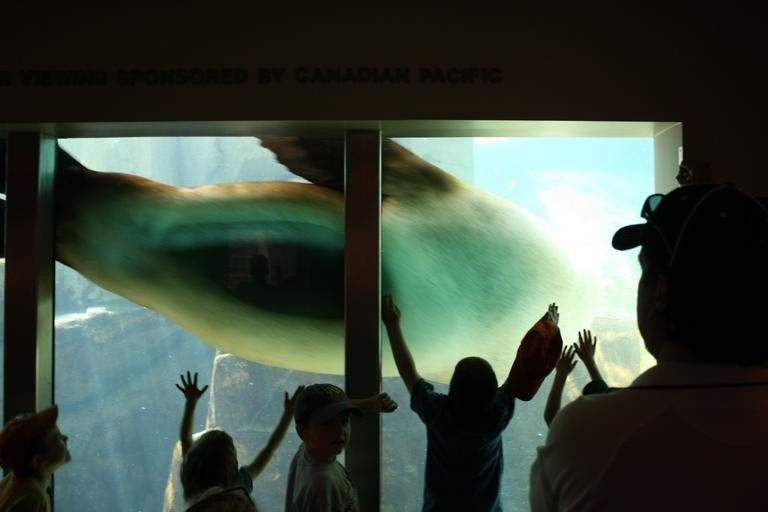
[
  {"xmin": 175, "ymin": 371, "xmax": 307, "ymax": 511},
  {"xmin": 0, "ymin": 404, "xmax": 73, "ymax": 512},
  {"xmin": 382, "ymin": 293, "xmax": 563, "ymax": 512},
  {"xmin": 527, "ymin": 181, "xmax": 766, "ymax": 511},
  {"xmin": 543, "ymin": 329, "xmax": 611, "ymax": 428},
  {"xmin": 282, "ymin": 384, "xmax": 398, "ymax": 511}
]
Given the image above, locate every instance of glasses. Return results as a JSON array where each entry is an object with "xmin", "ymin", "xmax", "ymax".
[{"xmin": 640, "ymin": 194, "xmax": 672, "ymax": 250}]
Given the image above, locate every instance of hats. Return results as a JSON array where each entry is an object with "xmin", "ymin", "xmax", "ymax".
[
  {"xmin": 1, "ymin": 405, "xmax": 58, "ymax": 467},
  {"xmin": 611, "ymin": 185, "xmax": 763, "ymax": 260},
  {"xmin": 293, "ymin": 383, "xmax": 360, "ymax": 425}
]
